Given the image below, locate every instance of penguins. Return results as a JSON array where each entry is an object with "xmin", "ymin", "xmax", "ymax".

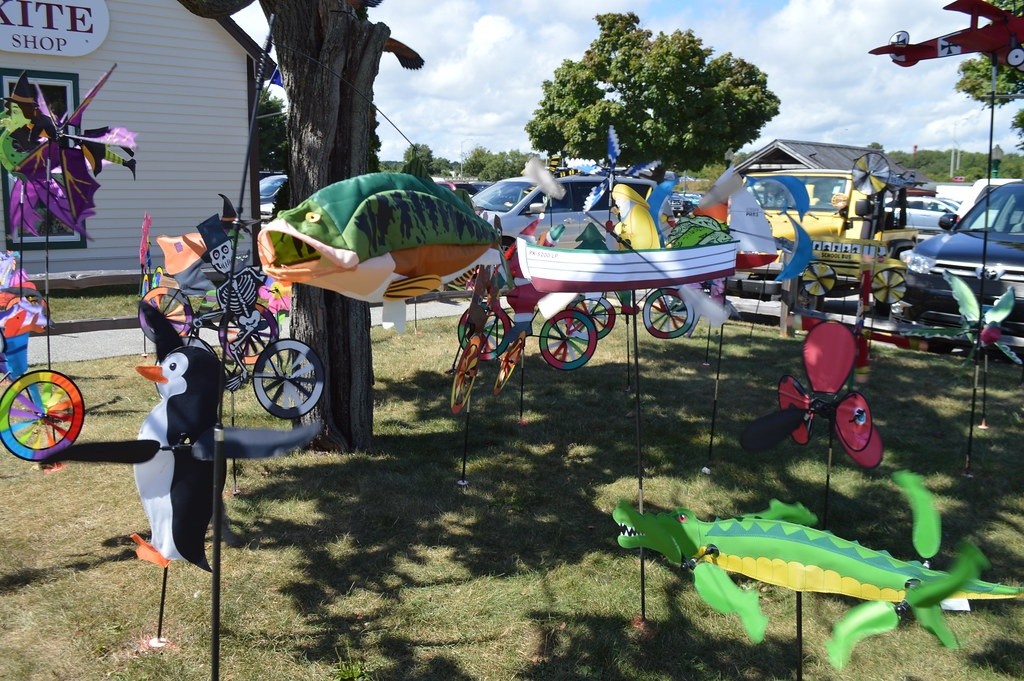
[{"xmin": 130, "ymin": 345, "xmax": 226, "ymax": 575}]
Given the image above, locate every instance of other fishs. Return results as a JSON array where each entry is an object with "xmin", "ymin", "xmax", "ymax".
[{"xmin": 612, "ymin": 498, "xmax": 1024, "ymax": 604}]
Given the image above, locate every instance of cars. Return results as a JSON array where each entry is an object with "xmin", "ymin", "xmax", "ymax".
[
  {"xmin": 666, "ymin": 169, "xmax": 1024, "ymax": 321},
  {"xmin": 899, "ymin": 180, "xmax": 1024, "ymax": 364},
  {"xmin": 430, "ymin": 177, "xmax": 493, "ymax": 196},
  {"xmin": 259, "ymin": 173, "xmax": 289, "ymax": 229}
]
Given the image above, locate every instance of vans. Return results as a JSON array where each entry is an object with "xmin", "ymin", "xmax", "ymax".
[{"xmin": 469, "ymin": 176, "xmax": 658, "ymax": 254}]
